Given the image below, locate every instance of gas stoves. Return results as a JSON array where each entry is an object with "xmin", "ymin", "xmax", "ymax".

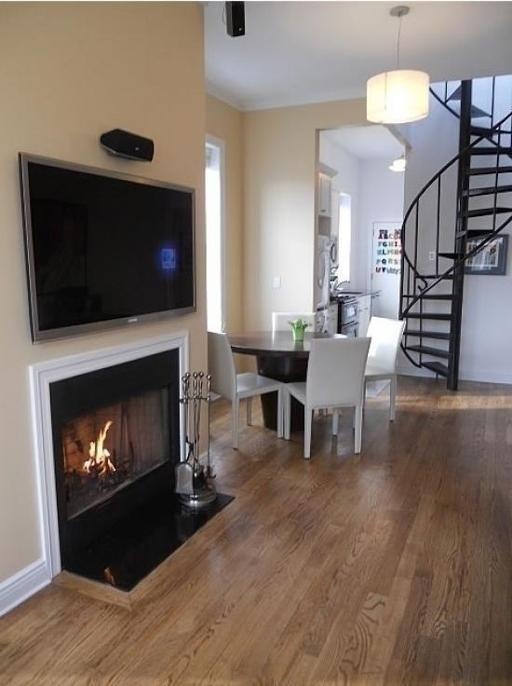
[
  {"xmin": 336, "ymin": 300, "xmax": 358, "ymax": 338},
  {"xmin": 334, "ymin": 296, "xmax": 353, "ymax": 303}
]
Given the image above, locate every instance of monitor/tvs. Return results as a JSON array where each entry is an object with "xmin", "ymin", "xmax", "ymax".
[{"xmin": 19, "ymin": 151, "xmax": 196, "ymax": 344}]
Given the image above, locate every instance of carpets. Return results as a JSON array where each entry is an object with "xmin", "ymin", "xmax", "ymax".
[{"xmin": 59, "ymin": 483, "xmax": 237, "ymax": 599}]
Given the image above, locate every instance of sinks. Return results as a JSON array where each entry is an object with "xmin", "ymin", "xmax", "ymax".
[{"xmin": 339, "ymin": 292, "xmax": 362, "ymax": 294}]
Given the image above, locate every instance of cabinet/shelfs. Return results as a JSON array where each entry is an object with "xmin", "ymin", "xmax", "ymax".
[
  {"xmin": 320, "ymin": 303, "xmax": 339, "ymax": 334},
  {"xmin": 358, "ymin": 294, "xmax": 372, "ymax": 336},
  {"xmin": 318, "ymin": 161, "xmax": 333, "ymax": 218},
  {"xmin": 320, "ymin": 191, "xmax": 340, "ymax": 238}
]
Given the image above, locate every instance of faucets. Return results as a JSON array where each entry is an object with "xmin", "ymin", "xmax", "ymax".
[{"xmin": 332, "ymin": 280, "xmax": 350, "ymax": 293}]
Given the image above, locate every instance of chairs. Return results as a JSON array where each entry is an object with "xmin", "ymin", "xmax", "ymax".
[
  {"xmin": 270, "ymin": 310, "xmax": 322, "ymax": 332},
  {"xmin": 281, "ymin": 329, "xmax": 370, "ymax": 460},
  {"xmin": 207, "ymin": 327, "xmax": 283, "ymax": 452},
  {"xmin": 358, "ymin": 316, "xmax": 409, "ymax": 427}
]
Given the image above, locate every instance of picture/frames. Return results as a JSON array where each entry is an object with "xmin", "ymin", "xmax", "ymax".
[{"xmin": 458, "ymin": 229, "xmax": 508, "ymax": 276}]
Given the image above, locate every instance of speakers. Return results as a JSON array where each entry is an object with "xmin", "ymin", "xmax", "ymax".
[
  {"xmin": 225, "ymin": 1, "xmax": 245, "ymax": 36},
  {"xmin": 99, "ymin": 128, "xmax": 154, "ymax": 161}
]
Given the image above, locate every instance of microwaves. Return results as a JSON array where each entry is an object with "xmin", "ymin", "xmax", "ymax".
[{"xmin": 330, "ymin": 237, "xmax": 338, "ymax": 269}]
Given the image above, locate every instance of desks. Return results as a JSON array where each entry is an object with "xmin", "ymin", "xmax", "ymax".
[{"xmin": 224, "ymin": 329, "xmax": 352, "ymax": 434}]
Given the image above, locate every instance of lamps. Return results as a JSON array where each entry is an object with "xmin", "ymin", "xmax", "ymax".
[
  {"xmin": 364, "ymin": 5, "xmax": 430, "ymax": 125},
  {"xmin": 386, "ymin": 152, "xmax": 406, "ymax": 173}
]
